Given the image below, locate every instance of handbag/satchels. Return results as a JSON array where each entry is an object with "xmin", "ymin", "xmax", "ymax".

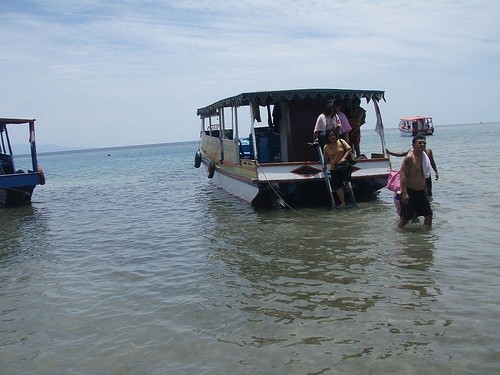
[{"xmin": 347, "ymin": 152, "xmax": 357, "ymax": 165}]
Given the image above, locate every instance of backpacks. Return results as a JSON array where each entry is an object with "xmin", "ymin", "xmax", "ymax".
[{"xmin": 386, "ymin": 169, "xmax": 404, "ymax": 193}]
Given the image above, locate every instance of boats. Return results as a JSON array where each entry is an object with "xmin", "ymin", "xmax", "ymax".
[
  {"xmin": 0, "ymin": 117, "xmax": 46, "ymax": 209},
  {"xmin": 397, "ymin": 115, "xmax": 435, "ymax": 137},
  {"xmin": 193, "ymin": 87, "xmax": 394, "ymax": 209}
]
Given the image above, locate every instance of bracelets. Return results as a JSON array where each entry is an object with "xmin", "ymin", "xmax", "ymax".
[{"xmin": 436, "ymin": 173, "xmax": 438, "ymax": 176}]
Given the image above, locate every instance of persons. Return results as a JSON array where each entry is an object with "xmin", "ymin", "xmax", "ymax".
[
  {"xmin": 383, "ymin": 120, "xmax": 439, "ymax": 229},
  {"xmin": 312, "ymin": 98, "xmax": 366, "ymax": 209}
]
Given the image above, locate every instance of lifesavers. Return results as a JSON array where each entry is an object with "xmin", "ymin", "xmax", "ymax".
[
  {"xmin": 194, "ymin": 151, "xmax": 202, "ymax": 169},
  {"xmin": 207, "ymin": 161, "xmax": 215, "ymax": 178}
]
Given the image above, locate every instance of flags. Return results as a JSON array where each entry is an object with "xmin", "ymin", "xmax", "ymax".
[{"xmin": 375, "ymin": 99, "xmax": 386, "ymax": 152}]
